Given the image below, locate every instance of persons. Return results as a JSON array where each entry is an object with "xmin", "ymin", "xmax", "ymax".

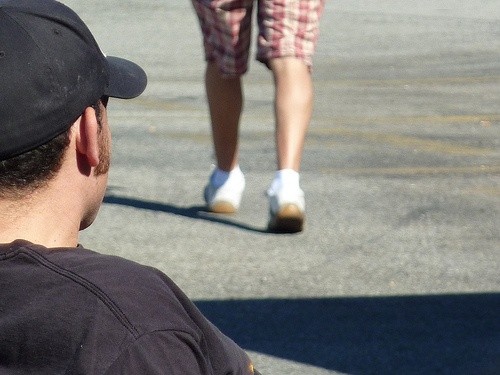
[
  {"xmin": 0, "ymin": 0, "xmax": 262, "ymax": 375},
  {"xmin": 191, "ymin": 0, "xmax": 326, "ymax": 230}
]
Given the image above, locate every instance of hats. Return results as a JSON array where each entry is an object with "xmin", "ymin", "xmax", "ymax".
[{"xmin": 0, "ymin": 0, "xmax": 148, "ymax": 158}]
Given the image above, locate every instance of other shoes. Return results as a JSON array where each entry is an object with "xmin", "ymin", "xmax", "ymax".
[
  {"xmin": 205, "ymin": 169, "xmax": 244, "ymax": 211},
  {"xmin": 267, "ymin": 169, "xmax": 305, "ymax": 230}
]
